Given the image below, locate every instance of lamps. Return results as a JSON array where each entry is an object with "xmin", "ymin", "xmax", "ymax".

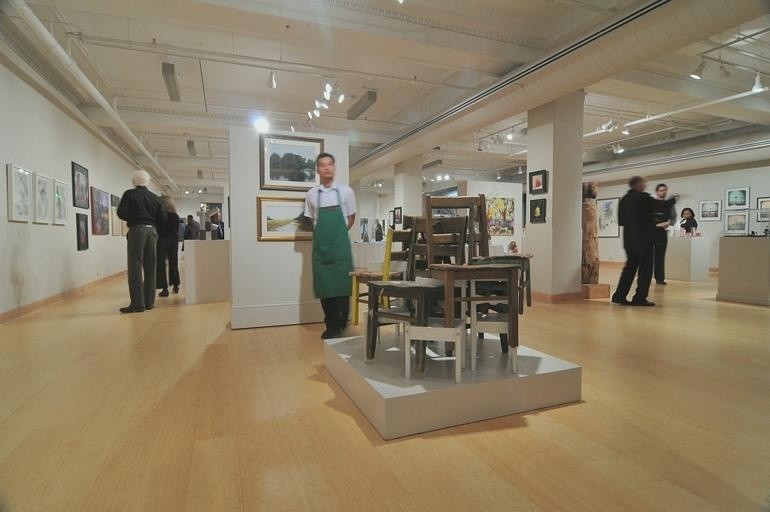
[
  {"xmin": 690, "ymin": 59, "xmax": 763, "ymax": 93},
  {"xmin": 496, "ymin": 165, "xmax": 523, "ymax": 179},
  {"xmin": 600, "ymin": 119, "xmax": 630, "ymax": 154},
  {"xmin": 306, "ymin": 83, "xmax": 345, "ymax": 119},
  {"xmin": 266, "ymin": 69, "xmax": 277, "ymax": 89},
  {"xmin": 476, "ymin": 126, "xmax": 527, "ymax": 151}
]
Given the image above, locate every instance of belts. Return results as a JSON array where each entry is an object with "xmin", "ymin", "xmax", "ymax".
[{"xmin": 135, "ymin": 224, "xmax": 153, "ymax": 228}]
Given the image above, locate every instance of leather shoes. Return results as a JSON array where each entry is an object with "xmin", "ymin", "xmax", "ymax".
[
  {"xmin": 632, "ymin": 296, "xmax": 655, "ymax": 306},
  {"xmin": 612, "ymin": 293, "xmax": 633, "ymax": 305}
]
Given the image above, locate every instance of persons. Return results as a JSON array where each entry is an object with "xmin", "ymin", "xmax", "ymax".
[
  {"xmin": 117, "ymin": 170, "xmax": 225, "ymax": 314},
  {"xmin": 302, "ymin": 152, "xmax": 358, "ymax": 338},
  {"xmin": 680, "ymin": 208, "xmax": 698, "ymax": 233},
  {"xmin": 611, "ymin": 176, "xmax": 678, "ymax": 306},
  {"xmin": 653, "ymin": 184, "xmax": 676, "ymax": 284}
]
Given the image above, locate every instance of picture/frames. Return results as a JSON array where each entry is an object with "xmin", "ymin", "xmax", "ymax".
[
  {"xmin": 257, "ymin": 196, "xmax": 313, "ymax": 240},
  {"xmin": 6, "ymin": 163, "xmax": 69, "ymax": 226},
  {"xmin": 529, "ymin": 198, "xmax": 546, "ymax": 224},
  {"xmin": 76, "ymin": 213, "xmax": 89, "ymax": 251},
  {"xmin": 259, "ymin": 133, "xmax": 324, "ymax": 191},
  {"xmin": 725, "ymin": 213, "xmax": 748, "ymax": 233},
  {"xmin": 726, "ymin": 186, "xmax": 750, "ymax": 210},
  {"xmin": 528, "ymin": 170, "xmax": 546, "ymax": 194},
  {"xmin": 757, "ymin": 197, "xmax": 770, "ymax": 222},
  {"xmin": 700, "ymin": 200, "xmax": 721, "ymax": 221},
  {"xmin": 71, "ymin": 161, "xmax": 89, "ymax": 208},
  {"xmin": 595, "ymin": 197, "xmax": 620, "ymax": 238}
]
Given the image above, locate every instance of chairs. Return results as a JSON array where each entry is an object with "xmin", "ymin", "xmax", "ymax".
[{"xmin": 346, "ymin": 193, "xmax": 532, "ymax": 384}]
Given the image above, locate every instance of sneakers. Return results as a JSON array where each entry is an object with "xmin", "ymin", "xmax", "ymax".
[
  {"xmin": 656, "ymin": 280, "xmax": 667, "ymax": 285},
  {"xmin": 145, "ymin": 304, "xmax": 155, "ymax": 310},
  {"xmin": 119, "ymin": 305, "xmax": 145, "ymax": 313},
  {"xmin": 320, "ymin": 324, "xmax": 349, "ymax": 338},
  {"xmin": 173, "ymin": 287, "xmax": 179, "ymax": 293},
  {"xmin": 159, "ymin": 288, "xmax": 169, "ymax": 297}
]
